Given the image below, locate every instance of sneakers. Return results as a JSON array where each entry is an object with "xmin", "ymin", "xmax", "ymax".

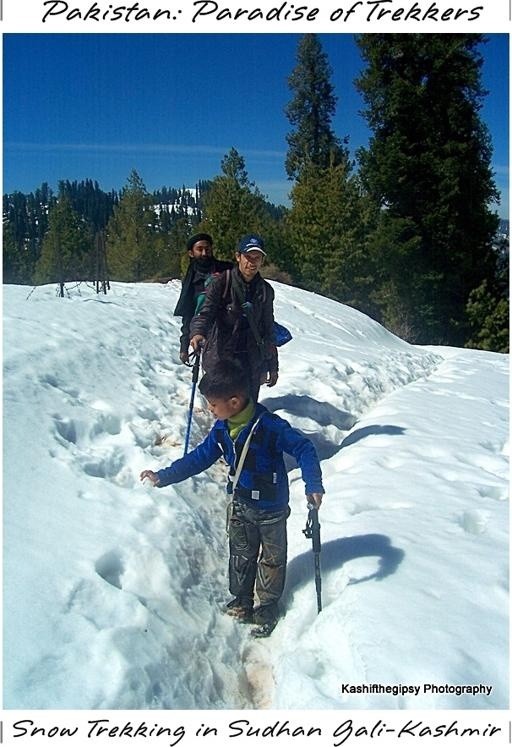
[
  {"xmin": 227, "ymin": 598, "xmax": 254, "ymax": 617},
  {"xmin": 243, "ymin": 604, "xmax": 279, "ymax": 623}
]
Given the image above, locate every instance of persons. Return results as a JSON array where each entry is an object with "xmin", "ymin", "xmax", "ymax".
[
  {"xmin": 187, "ymin": 234, "xmax": 279, "ymax": 402},
  {"xmin": 138, "ymin": 355, "xmax": 327, "ymax": 638},
  {"xmin": 172, "ymin": 232, "xmax": 234, "ymax": 364}
]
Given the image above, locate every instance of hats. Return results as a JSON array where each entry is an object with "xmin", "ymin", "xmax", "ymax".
[{"xmin": 238, "ymin": 235, "xmax": 267, "ymax": 256}]
